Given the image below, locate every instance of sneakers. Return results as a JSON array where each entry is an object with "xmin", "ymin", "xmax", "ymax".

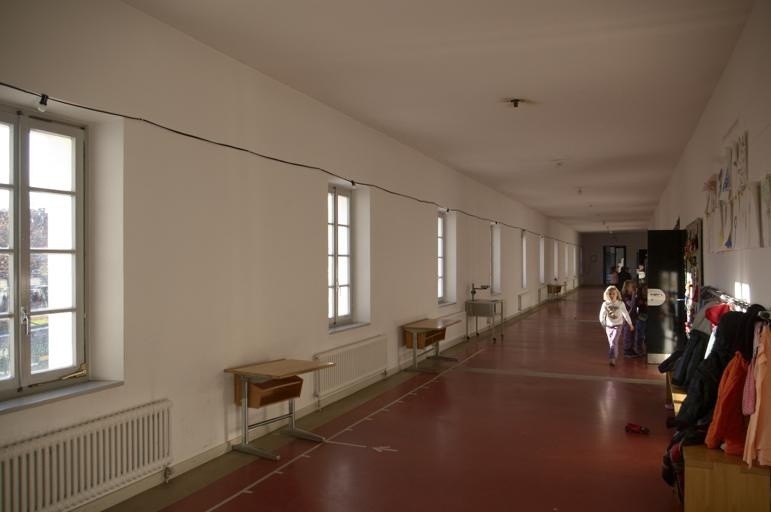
[{"xmin": 608, "ymin": 348, "xmax": 645, "ymax": 366}]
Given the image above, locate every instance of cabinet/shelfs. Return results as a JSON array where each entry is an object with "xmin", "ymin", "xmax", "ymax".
[{"xmin": 636, "ymin": 230, "xmax": 685, "ymax": 365}]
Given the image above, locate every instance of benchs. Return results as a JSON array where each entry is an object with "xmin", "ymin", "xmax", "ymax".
[{"xmin": 665, "ymin": 371, "xmax": 771, "ymax": 512}]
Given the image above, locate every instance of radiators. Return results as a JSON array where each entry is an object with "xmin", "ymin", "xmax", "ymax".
[
  {"xmin": 427, "ymin": 311, "xmax": 466, "ymax": 351},
  {"xmin": 487, "ymin": 292, "xmax": 530, "ymax": 324},
  {"xmin": 0, "ymin": 398, "xmax": 174, "ymax": 512},
  {"xmin": 538, "ymin": 287, "xmax": 548, "ymax": 302},
  {"xmin": 314, "ymin": 335, "xmax": 388, "ymax": 408},
  {"xmin": 565, "ymin": 278, "xmax": 579, "ymax": 291}
]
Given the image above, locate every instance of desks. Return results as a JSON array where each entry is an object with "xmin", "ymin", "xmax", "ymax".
[
  {"xmin": 224, "ymin": 358, "xmax": 336, "ymax": 461},
  {"xmin": 547, "ymin": 284, "xmax": 567, "ymax": 302},
  {"xmin": 465, "ymin": 299, "xmax": 504, "ymax": 342},
  {"xmin": 400, "ymin": 318, "xmax": 462, "ymax": 374}
]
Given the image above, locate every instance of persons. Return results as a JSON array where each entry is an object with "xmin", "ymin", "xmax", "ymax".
[
  {"xmin": 599, "ymin": 285, "xmax": 634, "ymax": 366},
  {"xmin": 621, "ymin": 279, "xmax": 642, "ymax": 358},
  {"xmin": 609, "ymin": 265, "xmax": 632, "ymax": 289}
]
